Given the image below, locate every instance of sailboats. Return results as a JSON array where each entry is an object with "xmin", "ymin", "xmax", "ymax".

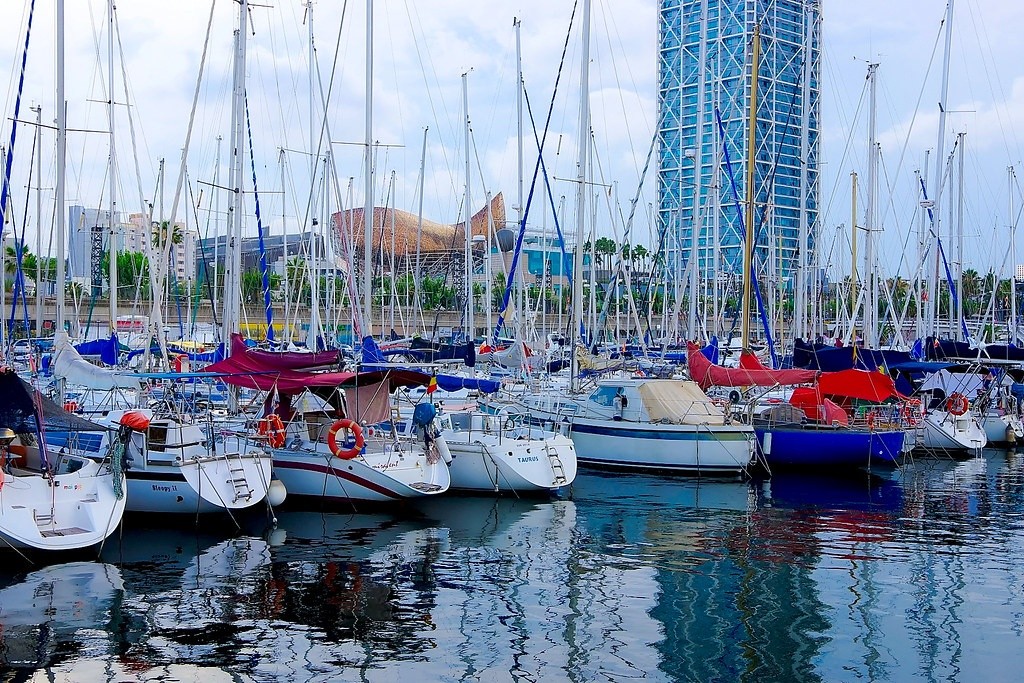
[{"xmin": 1, "ymin": 1, "xmax": 1024, "ymax": 570}]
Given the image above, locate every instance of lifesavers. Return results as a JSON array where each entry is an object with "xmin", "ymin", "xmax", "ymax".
[
  {"xmin": 904, "ymin": 398, "xmax": 925, "ymax": 425},
  {"xmin": 328, "ymin": 420, "xmax": 364, "ymax": 459},
  {"xmin": 947, "ymin": 391, "xmax": 969, "ymax": 416},
  {"xmin": 260, "ymin": 415, "xmax": 285, "ymax": 449}
]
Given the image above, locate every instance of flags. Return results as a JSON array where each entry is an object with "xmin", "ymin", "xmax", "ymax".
[
  {"xmin": 426, "ymin": 370, "xmax": 438, "ymax": 395},
  {"xmin": 877, "ymin": 363, "xmax": 889, "ymax": 376},
  {"xmin": 522, "ymin": 342, "xmax": 534, "ymax": 358}
]
